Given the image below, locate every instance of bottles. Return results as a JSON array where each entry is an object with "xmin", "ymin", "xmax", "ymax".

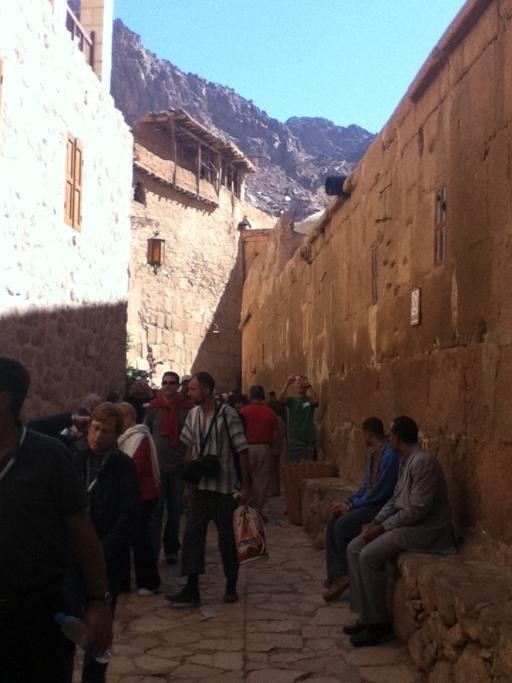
[{"xmin": 55, "ymin": 613, "xmax": 112, "ymax": 664}]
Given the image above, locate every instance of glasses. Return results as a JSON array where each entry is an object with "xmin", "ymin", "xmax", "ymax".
[{"xmin": 162, "ymin": 381, "xmax": 177, "ymax": 386}]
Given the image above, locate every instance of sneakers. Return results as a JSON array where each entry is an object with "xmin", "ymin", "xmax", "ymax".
[
  {"xmin": 120, "ymin": 539, "xmax": 240, "ymax": 610},
  {"xmin": 316, "ymin": 573, "xmax": 394, "ymax": 647}
]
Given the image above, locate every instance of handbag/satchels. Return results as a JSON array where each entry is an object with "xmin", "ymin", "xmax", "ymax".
[{"xmin": 232, "ymin": 504, "xmax": 271, "ymax": 564}]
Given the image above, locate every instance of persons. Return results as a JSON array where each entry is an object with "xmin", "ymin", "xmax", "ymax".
[
  {"xmin": 323, "ymin": 418, "xmax": 399, "ymax": 601},
  {"xmin": 343, "ymin": 416, "xmax": 459, "ymax": 646}
]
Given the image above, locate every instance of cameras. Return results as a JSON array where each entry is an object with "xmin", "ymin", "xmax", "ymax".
[{"xmin": 294, "ymin": 376, "xmax": 300, "ymax": 380}]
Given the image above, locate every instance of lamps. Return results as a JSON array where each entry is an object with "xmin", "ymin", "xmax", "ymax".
[{"xmin": 147, "ymin": 231, "xmax": 166, "ymax": 276}]
